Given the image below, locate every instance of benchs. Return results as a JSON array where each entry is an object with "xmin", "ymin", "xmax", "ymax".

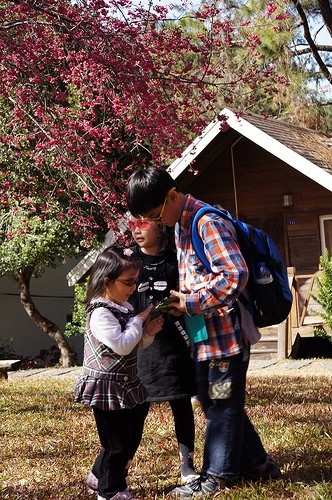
[{"xmin": 0, "ymin": 360, "xmax": 21, "ymax": 379}]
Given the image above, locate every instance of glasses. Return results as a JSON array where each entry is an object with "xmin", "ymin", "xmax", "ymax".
[
  {"xmin": 112, "ymin": 278, "xmax": 140, "ymax": 287},
  {"xmin": 128, "ymin": 219, "xmax": 155, "ymax": 231},
  {"xmin": 142, "ymin": 187, "xmax": 177, "ymax": 225}
]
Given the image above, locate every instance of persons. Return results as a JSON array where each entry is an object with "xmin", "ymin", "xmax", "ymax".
[
  {"xmin": 128, "ymin": 166, "xmax": 280, "ymax": 496},
  {"xmin": 125, "ymin": 209, "xmax": 200, "ymax": 483},
  {"xmin": 73, "ymin": 247, "xmax": 165, "ymax": 500}
]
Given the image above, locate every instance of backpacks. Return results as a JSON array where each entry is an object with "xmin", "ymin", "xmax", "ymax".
[{"xmin": 191, "ymin": 205, "xmax": 293, "ymax": 328}]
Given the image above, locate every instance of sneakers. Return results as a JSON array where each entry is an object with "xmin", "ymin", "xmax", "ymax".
[
  {"xmin": 172, "ymin": 473, "xmax": 234, "ymax": 498},
  {"xmin": 244, "ymin": 454, "xmax": 282, "ymax": 483}
]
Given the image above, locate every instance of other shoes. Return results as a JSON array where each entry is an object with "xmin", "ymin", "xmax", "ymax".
[
  {"xmin": 98, "ymin": 488, "xmax": 138, "ymax": 500},
  {"xmin": 86, "ymin": 471, "xmax": 100, "ymax": 491},
  {"xmin": 182, "ymin": 468, "xmax": 201, "ymax": 484}
]
